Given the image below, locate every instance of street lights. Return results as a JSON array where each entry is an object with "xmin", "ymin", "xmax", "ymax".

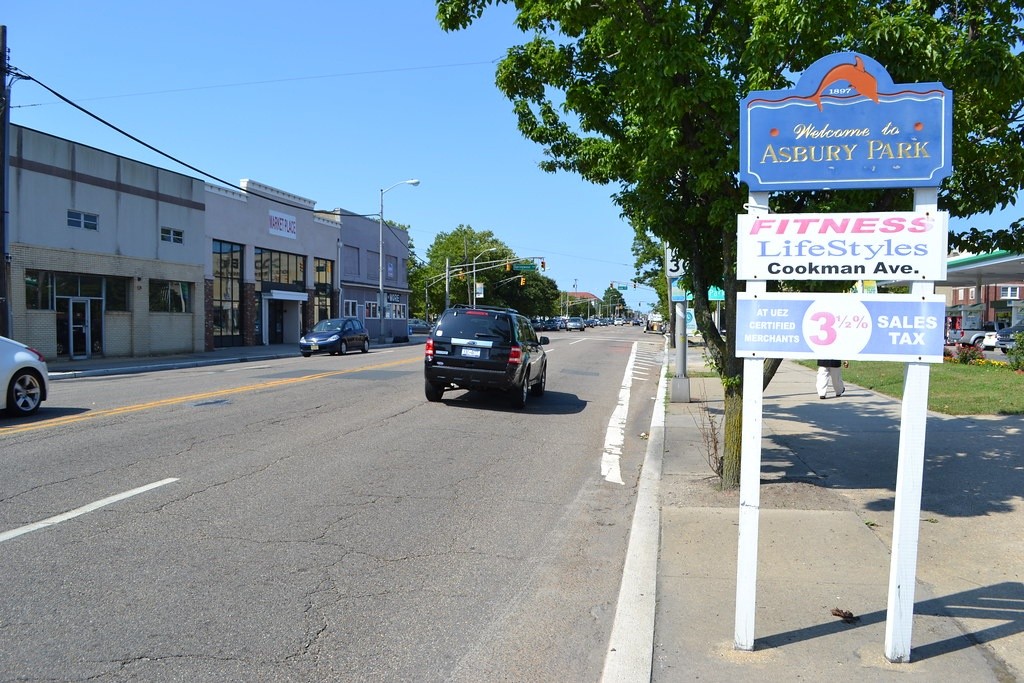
[
  {"xmin": 473, "ymin": 247, "xmax": 498, "ymax": 305},
  {"xmin": 376, "ymin": 178, "xmax": 421, "ymax": 343}
]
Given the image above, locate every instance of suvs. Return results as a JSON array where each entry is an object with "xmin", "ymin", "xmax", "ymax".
[{"xmin": 424, "ymin": 304, "xmax": 550, "ymax": 403}]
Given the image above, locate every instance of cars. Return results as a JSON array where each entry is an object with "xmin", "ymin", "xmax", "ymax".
[
  {"xmin": 565, "ymin": 317, "xmax": 585, "ymax": 332},
  {"xmin": 613, "ymin": 318, "xmax": 624, "ymax": 327},
  {"xmin": 299, "ymin": 316, "xmax": 370, "ymax": 358},
  {"xmin": 408, "ymin": 318, "xmax": 431, "ymax": 334},
  {"xmin": 996, "ymin": 320, "xmax": 1024, "ymax": 351},
  {"xmin": 531, "ymin": 318, "xmax": 614, "ymax": 331},
  {"xmin": 632, "ymin": 317, "xmax": 644, "ymax": 327},
  {"xmin": 0, "ymin": 334, "xmax": 52, "ymax": 420}
]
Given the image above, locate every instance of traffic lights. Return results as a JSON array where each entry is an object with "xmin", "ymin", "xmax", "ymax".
[
  {"xmin": 609, "ymin": 283, "xmax": 614, "ymax": 292},
  {"xmin": 633, "ymin": 283, "xmax": 637, "ymax": 291},
  {"xmin": 540, "ymin": 261, "xmax": 547, "ymax": 272}
]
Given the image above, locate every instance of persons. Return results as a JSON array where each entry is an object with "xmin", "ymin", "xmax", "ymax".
[{"xmin": 815, "ymin": 360, "xmax": 845, "ymax": 400}]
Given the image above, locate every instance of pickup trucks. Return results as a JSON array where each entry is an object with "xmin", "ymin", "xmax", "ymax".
[{"xmin": 949, "ymin": 319, "xmax": 1010, "ymax": 351}]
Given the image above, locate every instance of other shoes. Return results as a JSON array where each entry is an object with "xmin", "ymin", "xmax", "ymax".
[
  {"xmin": 836, "ymin": 387, "xmax": 845, "ymax": 397},
  {"xmin": 820, "ymin": 396, "xmax": 825, "ymax": 399}
]
